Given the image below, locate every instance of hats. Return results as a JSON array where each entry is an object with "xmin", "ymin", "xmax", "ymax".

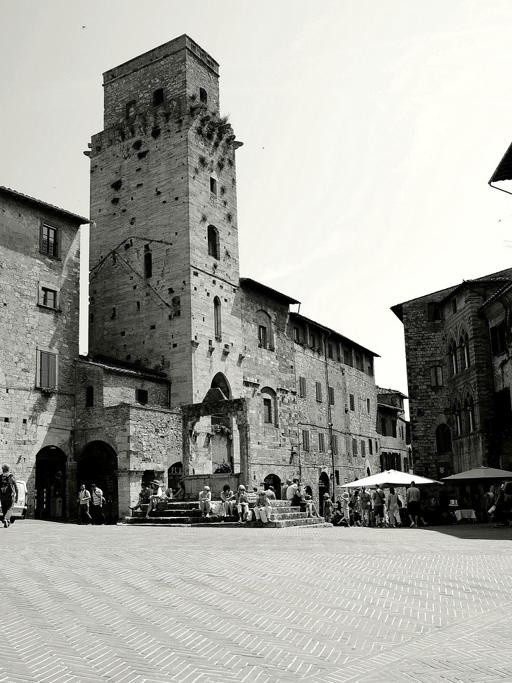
[{"xmin": 323, "ymin": 493, "xmax": 330, "ymax": 498}]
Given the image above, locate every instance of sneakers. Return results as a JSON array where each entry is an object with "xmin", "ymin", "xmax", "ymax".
[{"xmin": 2, "ymin": 519, "xmax": 10, "ymax": 528}]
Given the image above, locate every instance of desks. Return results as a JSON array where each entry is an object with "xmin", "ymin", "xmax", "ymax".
[{"xmin": 454, "ymin": 508, "xmax": 475, "ymax": 530}]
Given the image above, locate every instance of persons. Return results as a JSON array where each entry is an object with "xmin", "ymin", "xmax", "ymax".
[
  {"xmin": 128, "ymin": 482, "xmax": 149, "ymax": 510},
  {"xmin": 145, "ymin": 479, "xmax": 164, "ymax": 519},
  {"xmin": 488, "ymin": 488, "xmax": 509, "ymax": 528},
  {"xmin": 198, "ymin": 477, "xmax": 428, "ymax": 528},
  {"xmin": 87, "ymin": 483, "xmax": 106, "ymax": 525},
  {"xmin": 167, "ymin": 479, "xmax": 184, "ymax": 502},
  {"xmin": 0, "ymin": 466, "xmax": 18, "ymax": 528},
  {"xmin": 75, "ymin": 484, "xmax": 92, "ymax": 525}
]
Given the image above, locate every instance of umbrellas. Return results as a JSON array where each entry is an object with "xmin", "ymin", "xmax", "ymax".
[
  {"xmin": 439, "ymin": 466, "xmax": 512, "ymax": 480},
  {"xmin": 337, "ymin": 469, "xmax": 444, "ymax": 488}
]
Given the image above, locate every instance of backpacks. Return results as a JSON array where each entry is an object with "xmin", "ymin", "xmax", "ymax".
[{"xmin": 0, "ymin": 474, "xmax": 13, "ymax": 497}]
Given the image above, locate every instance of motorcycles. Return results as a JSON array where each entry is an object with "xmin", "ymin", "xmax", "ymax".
[{"xmin": 330, "ymin": 499, "xmax": 362, "ymax": 525}]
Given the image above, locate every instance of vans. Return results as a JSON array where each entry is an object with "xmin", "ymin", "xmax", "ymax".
[{"xmin": 0, "ymin": 479, "xmax": 28, "ymax": 525}]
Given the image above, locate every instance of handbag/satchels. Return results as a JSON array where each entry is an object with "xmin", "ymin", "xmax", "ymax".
[
  {"xmin": 156, "ymin": 499, "xmax": 169, "ymax": 511},
  {"xmin": 246, "ymin": 510, "xmax": 253, "ymax": 522},
  {"xmin": 348, "ymin": 500, "xmax": 356, "ymax": 509},
  {"xmin": 381, "ymin": 500, "xmax": 387, "ymax": 504},
  {"xmin": 259, "ymin": 507, "xmax": 268, "ymax": 523},
  {"xmin": 488, "ymin": 505, "xmax": 496, "ymax": 514},
  {"xmin": 397, "ymin": 495, "xmax": 402, "ymax": 508},
  {"xmin": 291, "ymin": 489, "xmax": 303, "ymax": 506},
  {"xmin": 305, "ymin": 496, "xmax": 310, "ymax": 500},
  {"xmin": 102, "ymin": 497, "xmax": 106, "ymax": 504}
]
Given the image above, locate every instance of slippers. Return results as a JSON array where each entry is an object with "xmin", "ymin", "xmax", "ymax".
[{"xmin": 410, "ymin": 521, "xmax": 415, "ymax": 528}]
